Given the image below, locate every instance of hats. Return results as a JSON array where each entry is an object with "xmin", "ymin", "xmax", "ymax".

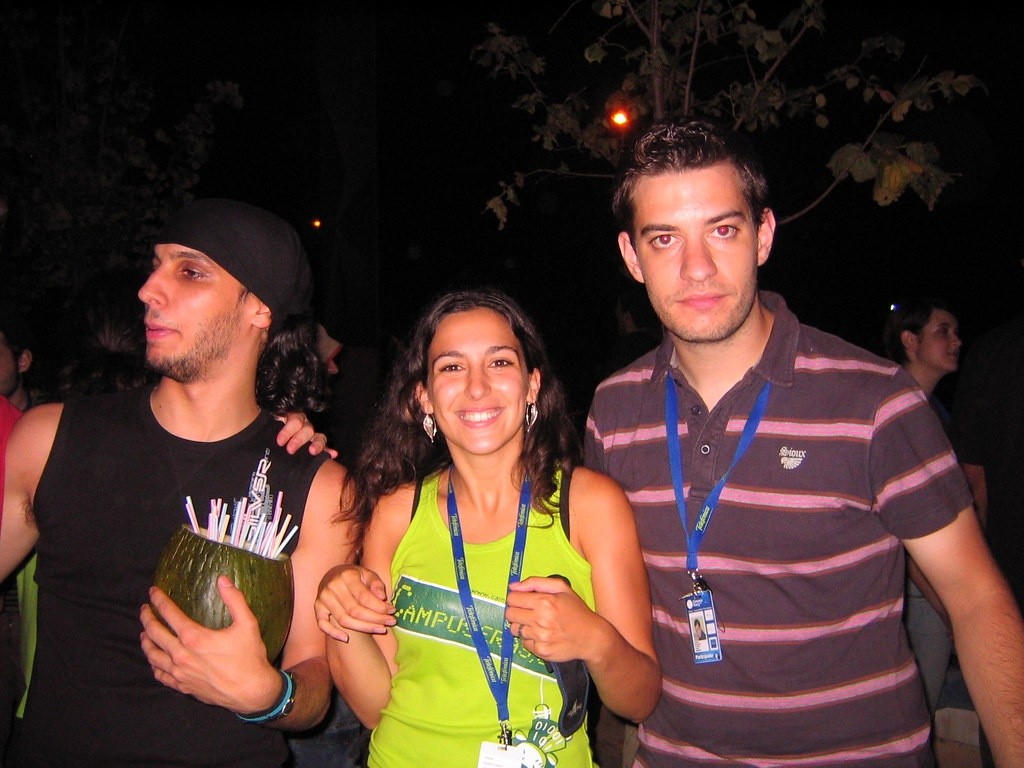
[{"xmin": 150, "ymin": 198, "xmax": 312, "ymax": 321}]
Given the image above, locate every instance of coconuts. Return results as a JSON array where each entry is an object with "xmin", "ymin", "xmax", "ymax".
[{"xmin": 152, "ymin": 522, "xmax": 294, "ymax": 669}]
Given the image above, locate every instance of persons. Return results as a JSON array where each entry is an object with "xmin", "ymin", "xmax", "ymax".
[
  {"xmin": 314, "ymin": 291, "xmax": 665, "ymax": 768},
  {"xmin": 880, "ymin": 254, "xmax": 1024, "ymax": 768},
  {"xmin": 581, "ymin": 120, "xmax": 1024, "ymax": 768},
  {"xmin": 583, "ymin": 282, "xmax": 661, "ymax": 401},
  {"xmin": 0, "ymin": 203, "xmax": 390, "ymax": 768},
  {"xmin": 694, "ymin": 619, "xmax": 706, "ymax": 640}
]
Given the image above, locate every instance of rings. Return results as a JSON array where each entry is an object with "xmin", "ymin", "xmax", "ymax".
[
  {"xmin": 518, "ymin": 624, "xmax": 525, "ymax": 636},
  {"xmin": 328, "ymin": 613, "xmax": 333, "ymax": 621}
]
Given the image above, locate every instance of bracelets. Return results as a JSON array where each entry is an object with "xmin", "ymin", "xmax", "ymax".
[{"xmin": 236, "ymin": 668, "xmax": 296, "ymax": 725}]
